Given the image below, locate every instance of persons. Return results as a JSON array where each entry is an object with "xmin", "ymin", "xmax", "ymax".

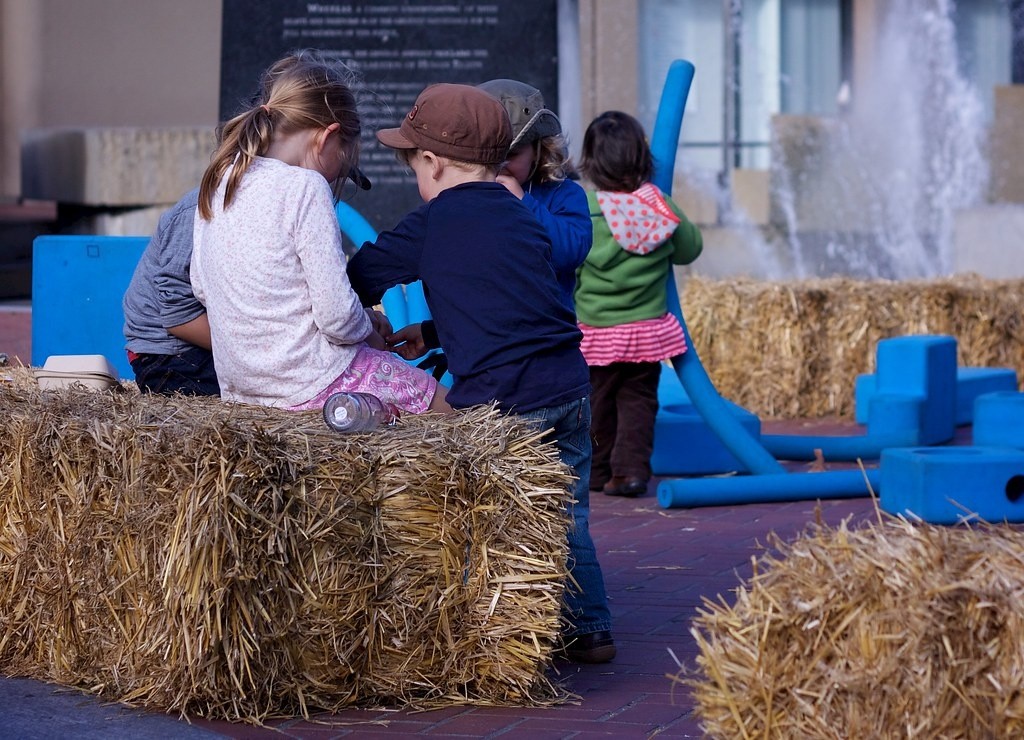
[
  {"xmin": 572, "ymin": 111, "xmax": 704, "ymax": 499},
  {"xmin": 123, "ymin": 51, "xmax": 618, "ymax": 684}
]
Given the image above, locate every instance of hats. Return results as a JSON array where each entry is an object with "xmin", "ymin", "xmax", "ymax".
[
  {"xmin": 376, "ymin": 82, "xmax": 513, "ymax": 164},
  {"xmin": 474, "ymin": 77, "xmax": 562, "ymax": 151},
  {"xmin": 338, "ymin": 159, "xmax": 372, "ymax": 193}
]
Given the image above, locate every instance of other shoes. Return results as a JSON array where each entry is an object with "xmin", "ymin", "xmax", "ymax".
[
  {"xmin": 603, "ymin": 475, "xmax": 647, "ymax": 496},
  {"xmin": 551, "ymin": 628, "xmax": 617, "ymax": 665},
  {"xmin": 588, "ymin": 472, "xmax": 609, "ymax": 492}
]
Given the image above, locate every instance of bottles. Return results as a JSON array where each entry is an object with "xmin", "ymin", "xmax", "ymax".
[{"xmin": 323, "ymin": 392, "xmax": 401, "ymax": 436}]
